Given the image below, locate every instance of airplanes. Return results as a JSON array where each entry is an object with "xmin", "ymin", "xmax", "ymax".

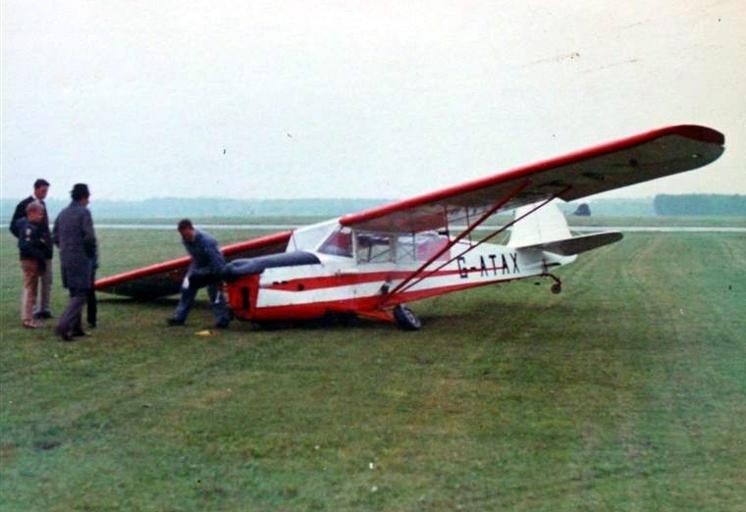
[{"xmin": 94, "ymin": 125, "xmax": 725, "ymax": 330}]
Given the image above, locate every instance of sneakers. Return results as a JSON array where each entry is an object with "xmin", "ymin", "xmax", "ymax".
[
  {"xmin": 167, "ymin": 318, "xmax": 184, "ymax": 327},
  {"xmin": 54, "ymin": 323, "xmax": 96, "ymax": 341},
  {"xmin": 23, "ymin": 311, "xmax": 52, "ymax": 328}
]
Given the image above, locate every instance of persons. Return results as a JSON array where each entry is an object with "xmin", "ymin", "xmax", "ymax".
[
  {"xmin": 13, "ymin": 179, "xmax": 54, "ymax": 320},
  {"xmin": 84, "ymin": 293, "xmax": 96, "ymax": 330},
  {"xmin": 165, "ymin": 218, "xmax": 229, "ymax": 333},
  {"xmin": 50, "ymin": 183, "xmax": 101, "ymax": 344},
  {"xmin": 12, "ymin": 201, "xmax": 47, "ymax": 329}
]
